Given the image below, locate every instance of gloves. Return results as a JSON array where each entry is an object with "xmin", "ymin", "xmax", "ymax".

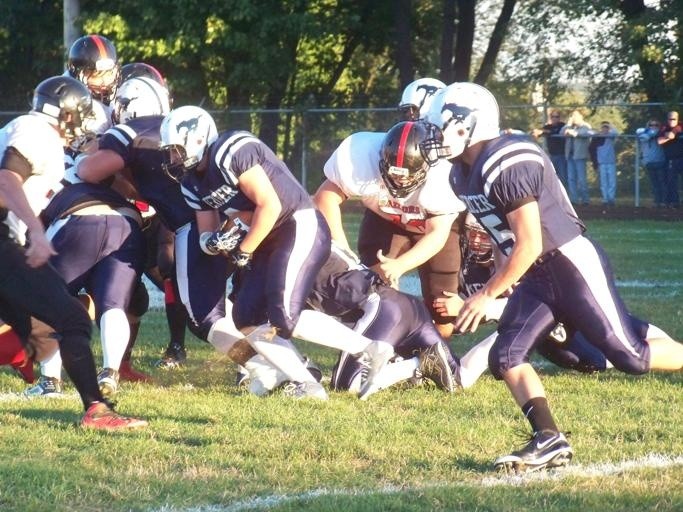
[{"xmin": 199, "ymin": 219, "xmax": 252, "ymax": 270}]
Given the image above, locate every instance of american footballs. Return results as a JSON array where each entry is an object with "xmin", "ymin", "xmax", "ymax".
[{"xmin": 222, "ymin": 210, "xmax": 253, "ymax": 260}]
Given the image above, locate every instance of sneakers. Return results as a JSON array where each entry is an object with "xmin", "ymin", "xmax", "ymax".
[
  {"xmin": 279, "ymin": 341, "xmax": 392, "ymax": 400},
  {"xmin": 154, "ymin": 342, "xmax": 184, "ymax": 367},
  {"xmin": 493, "ymin": 431, "xmax": 572, "ymax": 473},
  {"xmin": 26, "ymin": 377, "xmax": 61, "ymax": 395},
  {"xmin": 413, "ymin": 341, "xmax": 453, "ymax": 393},
  {"xmin": 81, "ymin": 367, "xmax": 147, "ymax": 429}
]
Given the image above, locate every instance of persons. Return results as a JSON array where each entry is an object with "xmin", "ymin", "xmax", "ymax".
[{"xmin": 1, "ymin": 35, "xmax": 683, "ymax": 472}]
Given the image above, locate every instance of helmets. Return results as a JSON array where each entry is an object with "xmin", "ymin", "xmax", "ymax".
[
  {"xmin": 33, "ymin": 34, "xmax": 171, "ymax": 138},
  {"xmin": 462, "ymin": 211, "xmax": 494, "ymax": 264},
  {"xmin": 378, "ymin": 78, "xmax": 500, "ymax": 198},
  {"xmin": 159, "ymin": 105, "xmax": 219, "ymax": 183}
]
